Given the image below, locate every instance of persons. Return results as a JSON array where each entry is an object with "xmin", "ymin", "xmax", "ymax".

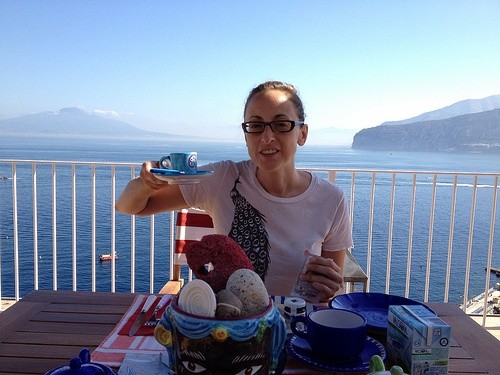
[{"xmin": 114, "ymin": 80, "xmax": 354, "ymax": 308}]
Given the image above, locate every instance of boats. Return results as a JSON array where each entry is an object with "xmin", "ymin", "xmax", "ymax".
[{"xmin": 99, "ymin": 251, "xmax": 118, "ymax": 261}]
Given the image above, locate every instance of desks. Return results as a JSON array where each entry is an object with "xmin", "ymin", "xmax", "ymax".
[{"xmin": 0, "ymin": 290, "xmax": 500, "ymax": 375}]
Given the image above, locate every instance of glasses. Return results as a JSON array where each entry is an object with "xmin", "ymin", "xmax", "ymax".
[{"xmin": 242, "ymin": 119, "xmax": 304, "ymax": 134}]
